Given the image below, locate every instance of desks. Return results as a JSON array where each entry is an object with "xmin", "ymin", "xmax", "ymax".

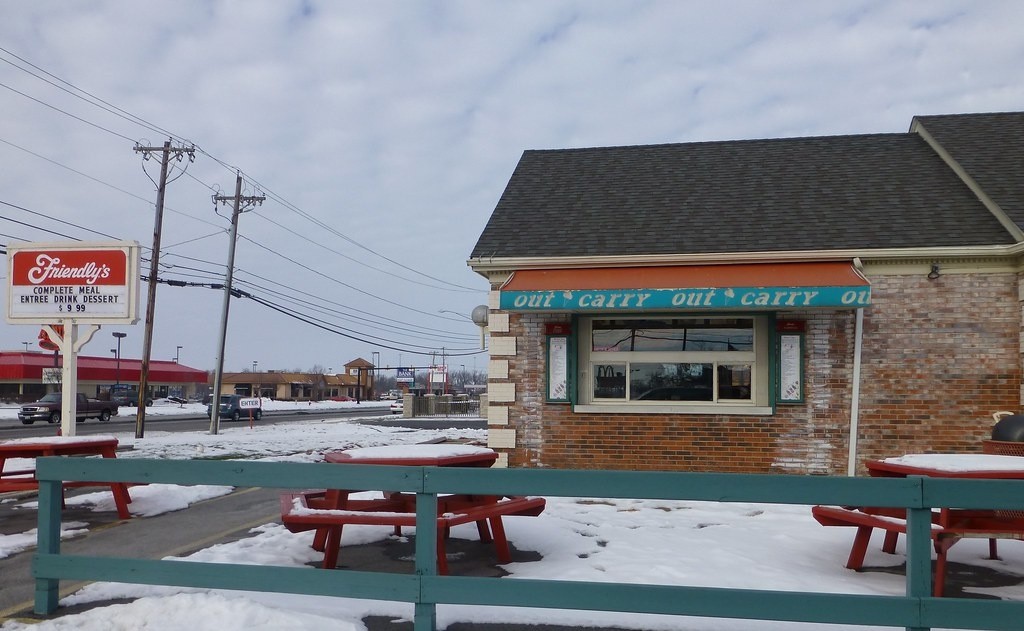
[
  {"xmin": 312, "ymin": 446, "xmax": 511, "ymax": 576},
  {"xmin": 0, "ymin": 436, "xmax": 132, "ymax": 518},
  {"xmin": 846, "ymin": 461, "xmax": 1024, "ymax": 597}
]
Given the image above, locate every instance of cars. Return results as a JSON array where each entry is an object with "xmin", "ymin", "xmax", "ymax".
[
  {"xmin": 633, "ymin": 383, "xmax": 707, "ymax": 400},
  {"xmin": 331, "ymin": 395, "xmax": 356, "ymax": 402},
  {"xmin": 390, "ymin": 398, "xmax": 404, "ymax": 414},
  {"xmin": 380, "ymin": 392, "xmax": 399, "ymax": 400}
]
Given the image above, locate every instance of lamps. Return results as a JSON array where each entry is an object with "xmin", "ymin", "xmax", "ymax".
[
  {"xmin": 928, "ymin": 265, "xmax": 938, "ymax": 279},
  {"xmin": 471, "ymin": 305, "xmax": 491, "ymax": 349}
]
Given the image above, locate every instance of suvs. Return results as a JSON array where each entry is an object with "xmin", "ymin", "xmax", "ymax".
[
  {"xmin": 112, "ymin": 390, "xmax": 154, "ymax": 408},
  {"xmin": 207, "ymin": 394, "xmax": 262, "ymax": 422}
]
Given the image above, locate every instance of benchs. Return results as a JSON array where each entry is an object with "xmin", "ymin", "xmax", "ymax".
[
  {"xmin": 0, "ymin": 468, "xmax": 149, "ymax": 492},
  {"xmin": 812, "ymin": 505, "xmax": 1024, "ymax": 554},
  {"xmin": 280, "ymin": 488, "xmax": 546, "ymax": 533}
]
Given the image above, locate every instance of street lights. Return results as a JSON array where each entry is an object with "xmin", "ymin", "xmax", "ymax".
[
  {"xmin": 171, "ymin": 357, "xmax": 178, "ymax": 363},
  {"xmin": 252, "ymin": 360, "xmax": 257, "ymax": 373},
  {"xmin": 177, "ymin": 345, "xmax": 183, "ymax": 363},
  {"xmin": 111, "ymin": 331, "xmax": 127, "ymax": 383},
  {"xmin": 372, "ymin": 352, "xmax": 379, "ymax": 382},
  {"xmin": 460, "ymin": 364, "xmax": 465, "ymax": 372},
  {"xmin": 21, "ymin": 341, "xmax": 33, "ymax": 351},
  {"xmin": 111, "ymin": 349, "xmax": 116, "ymax": 358}
]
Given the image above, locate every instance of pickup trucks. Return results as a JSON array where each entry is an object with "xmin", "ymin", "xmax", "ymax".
[{"xmin": 18, "ymin": 392, "xmax": 119, "ymax": 424}]
